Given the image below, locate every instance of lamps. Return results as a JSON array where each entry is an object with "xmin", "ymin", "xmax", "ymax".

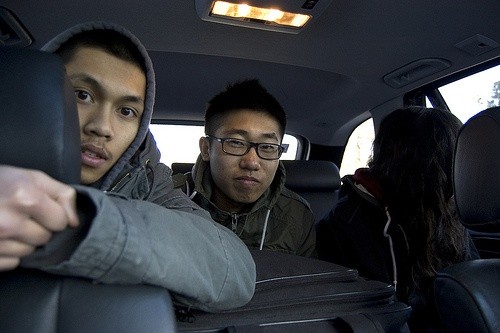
[{"xmin": 195, "ymin": 0, "xmax": 335, "ymax": 36}]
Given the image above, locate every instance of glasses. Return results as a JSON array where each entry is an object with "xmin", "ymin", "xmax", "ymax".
[{"xmin": 206, "ymin": 133, "xmax": 285, "ymax": 160}]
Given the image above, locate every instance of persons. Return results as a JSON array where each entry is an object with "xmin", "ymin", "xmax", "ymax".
[
  {"xmin": 315, "ymin": 105, "xmax": 481, "ymax": 323},
  {"xmin": 172, "ymin": 77, "xmax": 317, "ymax": 256},
  {"xmin": 1, "ymin": 20, "xmax": 257, "ymax": 312}
]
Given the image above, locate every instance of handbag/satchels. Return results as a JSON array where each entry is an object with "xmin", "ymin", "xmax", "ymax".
[{"xmin": 173, "ymin": 246, "xmax": 410, "ymax": 333}]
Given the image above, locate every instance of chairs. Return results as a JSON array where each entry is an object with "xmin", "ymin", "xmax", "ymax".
[
  {"xmin": 432, "ymin": 106, "xmax": 500, "ymax": 333},
  {"xmin": 0, "ymin": 46, "xmax": 177, "ymax": 333},
  {"xmin": 172, "ymin": 160, "xmax": 340, "ymax": 224}
]
